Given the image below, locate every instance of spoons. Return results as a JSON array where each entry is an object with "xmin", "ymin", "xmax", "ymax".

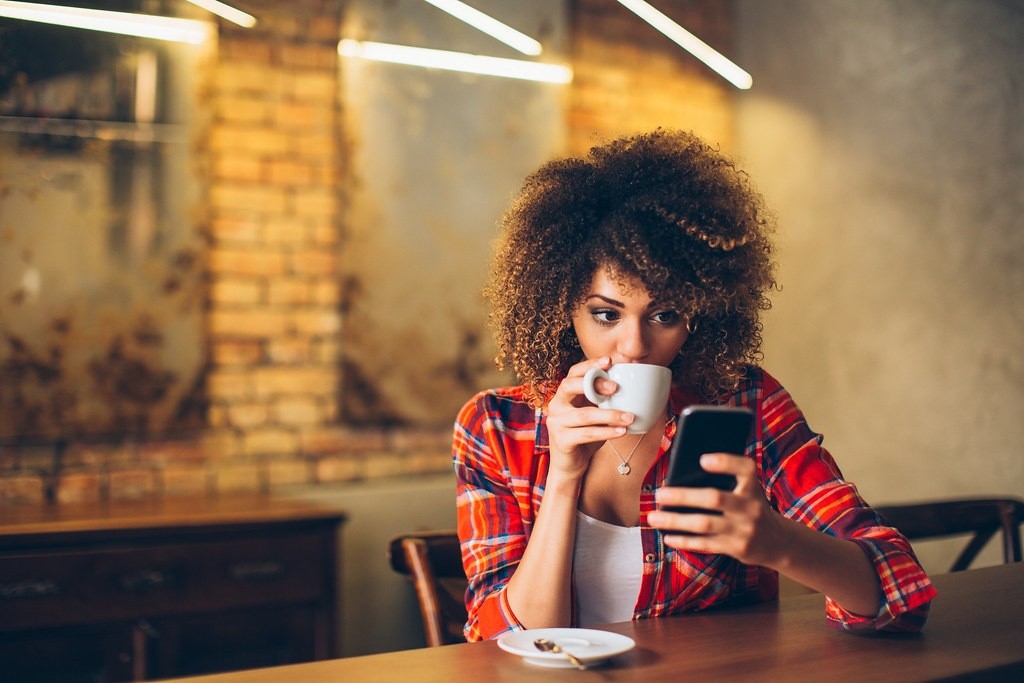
[{"xmin": 534, "ymin": 638, "xmax": 587, "ymax": 671}]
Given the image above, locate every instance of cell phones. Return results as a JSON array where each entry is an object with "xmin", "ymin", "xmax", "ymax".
[{"xmin": 658, "ymin": 404, "xmax": 756, "ymax": 536}]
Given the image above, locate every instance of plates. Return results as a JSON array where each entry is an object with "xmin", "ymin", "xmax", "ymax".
[{"xmin": 497, "ymin": 627, "xmax": 636, "ymax": 671}]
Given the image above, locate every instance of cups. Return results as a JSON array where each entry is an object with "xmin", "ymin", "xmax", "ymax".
[{"xmin": 584, "ymin": 363, "xmax": 673, "ymax": 435}]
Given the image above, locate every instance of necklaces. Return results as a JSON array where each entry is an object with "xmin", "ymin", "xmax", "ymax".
[{"xmin": 608, "ymin": 435, "xmax": 649, "ymax": 476}]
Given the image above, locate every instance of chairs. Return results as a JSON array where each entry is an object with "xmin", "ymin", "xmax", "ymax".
[
  {"xmin": 874, "ymin": 498, "xmax": 1024, "ymax": 572},
  {"xmin": 386, "ymin": 531, "xmax": 465, "ymax": 647}
]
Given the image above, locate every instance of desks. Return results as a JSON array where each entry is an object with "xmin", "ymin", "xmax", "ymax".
[
  {"xmin": 0, "ymin": 494, "xmax": 351, "ymax": 683},
  {"xmin": 166, "ymin": 560, "xmax": 1024, "ymax": 683}
]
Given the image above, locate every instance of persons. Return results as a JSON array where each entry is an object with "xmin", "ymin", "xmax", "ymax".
[{"xmin": 452, "ymin": 128, "xmax": 935, "ymax": 642}]
